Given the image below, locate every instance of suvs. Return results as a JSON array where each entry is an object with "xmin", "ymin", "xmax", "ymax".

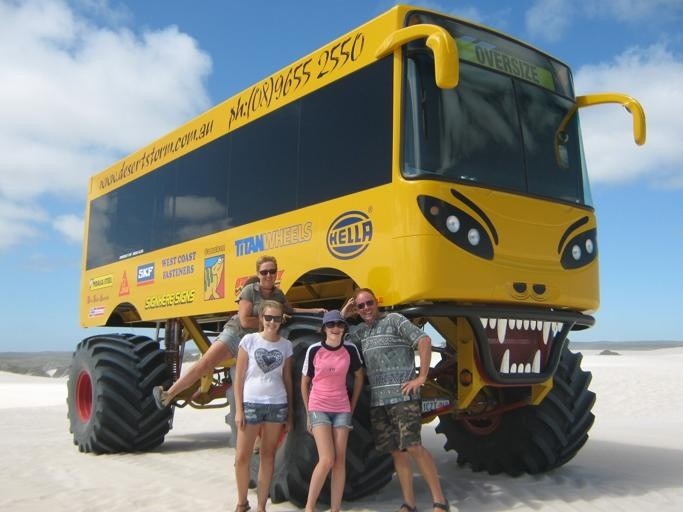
[{"xmin": 64, "ymin": 5, "xmax": 647, "ymax": 505}]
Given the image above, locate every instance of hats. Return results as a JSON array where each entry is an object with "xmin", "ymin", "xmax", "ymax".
[{"xmin": 321, "ymin": 310, "xmax": 350, "ymax": 337}]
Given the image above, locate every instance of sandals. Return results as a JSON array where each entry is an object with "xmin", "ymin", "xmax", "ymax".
[
  {"xmin": 400, "ymin": 504, "xmax": 417, "ymax": 512},
  {"xmin": 234, "ymin": 501, "xmax": 250, "ymax": 512},
  {"xmin": 433, "ymin": 498, "xmax": 449, "ymax": 512}
]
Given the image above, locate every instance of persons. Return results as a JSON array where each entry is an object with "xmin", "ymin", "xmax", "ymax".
[
  {"xmin": 229, "ymin": 300, "xmax": 296, "ymax": 512},
  {"xmin": 300, "ymin": 305, "xmax": 367, "ymax": 512},
  {"xmin": 340, "ymin": 288, "xmax": 450, "ymax": 511},
  {"xmin": 151, "ymin": 255, "xmax": 330, "ymax": 408}
]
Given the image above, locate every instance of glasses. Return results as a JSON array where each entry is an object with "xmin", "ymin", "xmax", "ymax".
[
  {"xmin": 263, "ymin": 315, "xmax": 282, "ymax": 322},
  {"xmin": 259, "ymin": 269, "xmax": 277, "ymax": 276},
  {"xmin": 326, "ymin": 321, "xmax": 345, "ymax": 328},
  {"xmin": 356, "ymin": 300, "xmax": 373, "ymax": 309}
]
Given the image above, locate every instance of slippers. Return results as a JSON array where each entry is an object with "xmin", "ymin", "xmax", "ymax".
[{"xmin": 153, "ymin": 386, "xmax": 166, "ymax": 410}]
